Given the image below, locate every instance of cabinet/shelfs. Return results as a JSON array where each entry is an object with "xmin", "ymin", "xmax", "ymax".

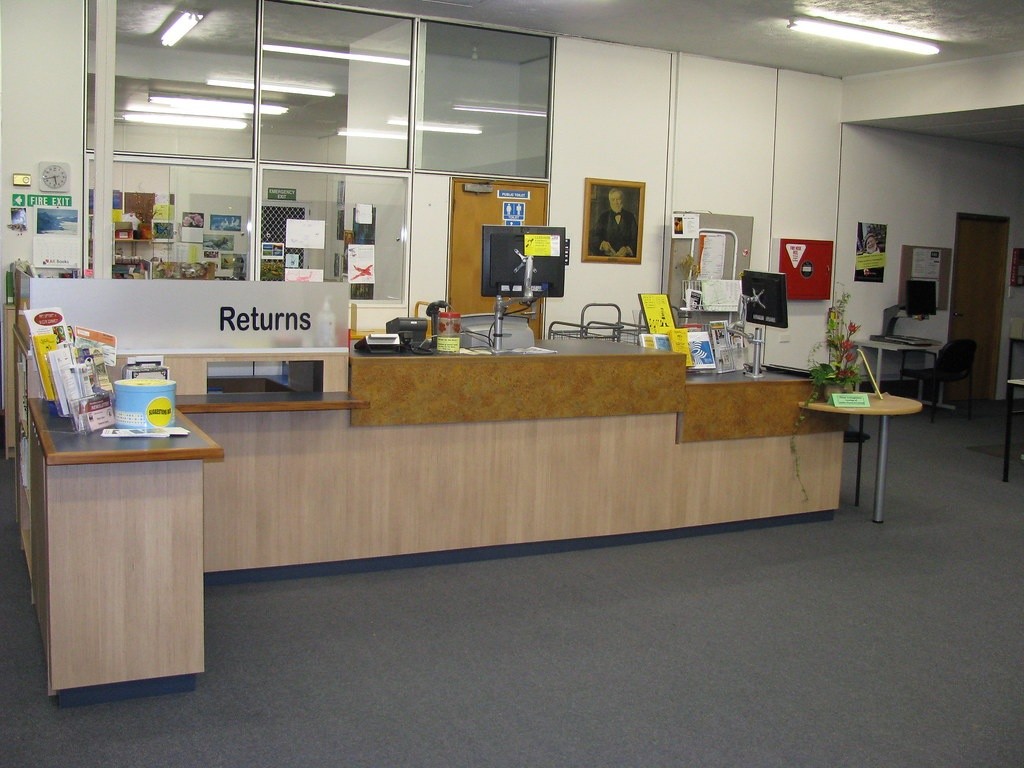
[
  {"xmin": 11, "ymin": 324, "xmax": 36, "ymax": 605},
  {"xmin": 680, "ymin": 280, "xmax": 744, "ymax": 349}
]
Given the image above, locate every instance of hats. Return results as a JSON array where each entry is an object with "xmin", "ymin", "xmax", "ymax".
[{"xmin": 864, "ymin": 233, "xmax": 876, "ymax": 248}]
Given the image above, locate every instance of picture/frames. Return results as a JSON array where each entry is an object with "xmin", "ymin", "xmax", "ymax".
[{"xmin": 582, "ymin": 177, "xmax": 647, "ymax": 265}]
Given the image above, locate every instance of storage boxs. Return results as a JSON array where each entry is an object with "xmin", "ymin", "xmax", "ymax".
[{"xmin": 115, "ymin": 222, "xmax": 132, "ymax": 239}]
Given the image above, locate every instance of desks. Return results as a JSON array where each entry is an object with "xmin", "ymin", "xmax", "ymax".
[
  {"xmin": 852, "ymin": 337, "xmax": 956, "ymax": 410},
  {"xmin": 1002, "ymin": 379, "xmax": 1024, "ymax": 481},
  {"xmin": 27, "ymin": 397, "xmax": 225, "ymax": 695},
  {"xmin": 799, "ymin": 392, "xmax": 923, "ymax": 523}
]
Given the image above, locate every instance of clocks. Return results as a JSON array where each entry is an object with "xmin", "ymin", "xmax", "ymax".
[{"xmin": 39, "ymin": 160, "xmax": 71, "ymax": 193}]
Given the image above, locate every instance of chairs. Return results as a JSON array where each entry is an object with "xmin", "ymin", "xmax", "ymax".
[{"xmin": 898, "ymin": 338, "xmax": 977, "ymax": 423}]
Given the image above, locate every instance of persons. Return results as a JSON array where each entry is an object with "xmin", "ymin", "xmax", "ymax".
[
  {"xmin": 861, "ymin": 234, "xmax": 885, "ymax": 277},
  {"xmin": 589, "ymin": 187, "xmax": 638, "ymax": 258}
]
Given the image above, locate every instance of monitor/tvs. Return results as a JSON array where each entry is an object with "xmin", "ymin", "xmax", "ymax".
[
  {"xmin": 481, "ymin": 225, "xmax": 566, "ymax": 354},
  {"xmin": 726, "ymin": 269, "xmax": 788, "ymax": 379},
  {"xmin": 870, "ymin": 280, "xmax": 936, "ymax": 345}
]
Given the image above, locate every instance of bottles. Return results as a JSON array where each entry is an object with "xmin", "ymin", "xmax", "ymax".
[{"xmin": 437, "ymin": 311, "xmax": 461, "ymax": 356}]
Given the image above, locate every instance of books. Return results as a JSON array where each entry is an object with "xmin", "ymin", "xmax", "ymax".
[
  {"xmin": 33, "ymin": 333, "xmax": 94, "ymax": 414},
  {"xmin": 638, "ymin": 332, "xmax": 716, "ymax": 372}
]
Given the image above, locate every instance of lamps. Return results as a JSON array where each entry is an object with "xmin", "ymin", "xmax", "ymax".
[
  {"xmin": 262, "ymin": 39, "xmax": 411, "ymax": 66},
  {"xmin": 788, "ymin": 16, "xmax": 941, "ymax": 56},
  {"xmin": 147, "ymin": 90, "xmax": 289, "ymax": 116},
  {"xmin": 161, "ymin": 10, "xmax": 204, "ymax": 46}
]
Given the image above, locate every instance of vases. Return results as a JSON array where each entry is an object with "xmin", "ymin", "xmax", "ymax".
[
  {"xmin": 138, "ymin": 223, "xmax": 152, "ymax": 239},
  {"xmin": 824, "ymin": 384, "xmax": 853, "ymax": 400}
]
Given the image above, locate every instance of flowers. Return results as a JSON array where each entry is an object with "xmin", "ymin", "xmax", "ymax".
[
  {"xmin": 790, "ymin": 291, "xmax": 862, "ymax": 503},
  {"xmin": 130, "ymin": 189, "xmax": 166, "ymax": 223}
]
